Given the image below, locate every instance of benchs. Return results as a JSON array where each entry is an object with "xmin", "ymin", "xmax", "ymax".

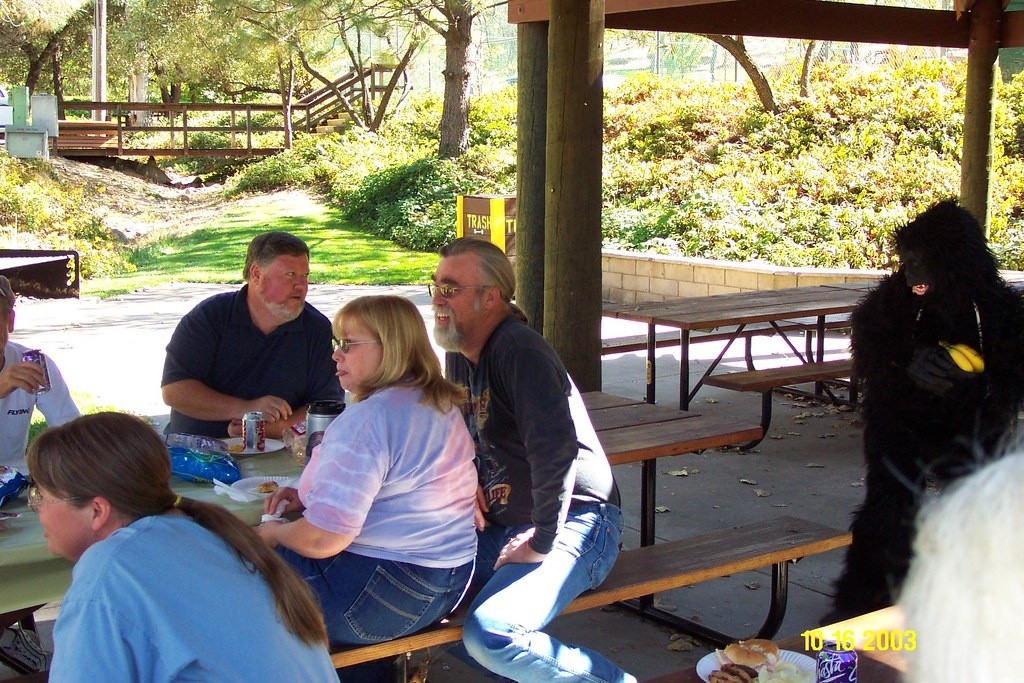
[
  {"xmin": 335, "ymin": 514, "xmax": 854, "ymax": 672},
  {"xmin": 601, "ymin": 312, "xmax": 868, "ymax": 392},
  {"xmin": 48, "ymin": 121, "xmax": 119, "ymax": 149}
]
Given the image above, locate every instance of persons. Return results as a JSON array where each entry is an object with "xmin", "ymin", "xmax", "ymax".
[
  {"xmin": 162, "ymin": 232, "xmax": 345, "ymax": 438},
  {"xmin": 260, "ymin": 295, "xmax": 479, "ymax": 683},
  {"xmin": 431, "ymin": 237, "xmax": 637, "ymax": 683},
  {"xmin": 905, "ymin": 454, "xmax": 1024, "ymax": 683},
  {"xmin": 0, "ymin": 276, "xmax": 81, "ymax": 478},
  {"xmin": 28, "ymin": 412, "xmax": 339, "ymax": 683}
]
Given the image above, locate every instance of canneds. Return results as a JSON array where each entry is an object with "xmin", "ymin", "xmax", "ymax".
[
  {"xmin": 242, "ymin": 410, "xmax": 265, "ymax": 452},
  {"xmin": 815, "ymin": 640, "xmax": 858, "ymax": 683},
  {"xmin": 21, "ymin": 348, "xmax": 52, "ymax": 394}
]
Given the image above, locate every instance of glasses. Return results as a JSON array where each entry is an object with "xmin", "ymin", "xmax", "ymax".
[
  {"xmin": 427, "ymin": 282, "xmax": 490, "ymax": 298},
  {"xmin": 29, "ymin": 485, "xmax": 81, "ymax": 513},
  {"xmin": 331, "ymin": 338, "xmax": 382, "ymax": 353}
]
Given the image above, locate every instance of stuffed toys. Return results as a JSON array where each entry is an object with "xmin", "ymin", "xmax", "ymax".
[{"xmin": 821, "ymin": 200, "xmax": 1023, "ymax": 623}]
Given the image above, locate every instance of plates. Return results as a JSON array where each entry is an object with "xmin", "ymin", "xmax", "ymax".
[
  {"xmin": 219, "ymin": 437, "xmax": 287, "ymax": 454},
  {"xmin": 696, "ymin": 649, "xmax": 819, "ymax": 683},
  {"xmin": 231, "ymin": 476, "xmax": 300, "ymax": 500}
]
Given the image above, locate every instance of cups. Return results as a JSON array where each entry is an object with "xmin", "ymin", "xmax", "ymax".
[{"xmin": 305, "ymin": 399, "xmax": 346, "ymax": 468}]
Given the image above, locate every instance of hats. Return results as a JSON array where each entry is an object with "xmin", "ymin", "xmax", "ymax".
[{"xmin": 0, "ymin": 275, "xmax": 15, "ymax": 309}]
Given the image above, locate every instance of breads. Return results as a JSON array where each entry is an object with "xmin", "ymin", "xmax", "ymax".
[
  {"xmin": 258, "ymin": 480, "xmax": 278, "ymax": 493},
  {"xmin": 723, "ymin": 637, "xmax": 780, "ymax": 667},
  {"xmin": 288, "ymin": 427, "xmax": 306, "ymax": 466},
  {"xmin": 229, "ymin": 443, "xmax": 243, "ymax": 452}
]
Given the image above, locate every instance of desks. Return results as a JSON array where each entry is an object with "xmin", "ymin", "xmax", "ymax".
[
  {"xmin": 810, "ymin": 282, "xmax": 889, "ymax": 405},
  {"xmin": 0, "ymin": 390, "xmax": 765, "ymax": 625},
  {"xmin": 602, "ymin": 286, "xmax": 872, "ymax": 411}
]
